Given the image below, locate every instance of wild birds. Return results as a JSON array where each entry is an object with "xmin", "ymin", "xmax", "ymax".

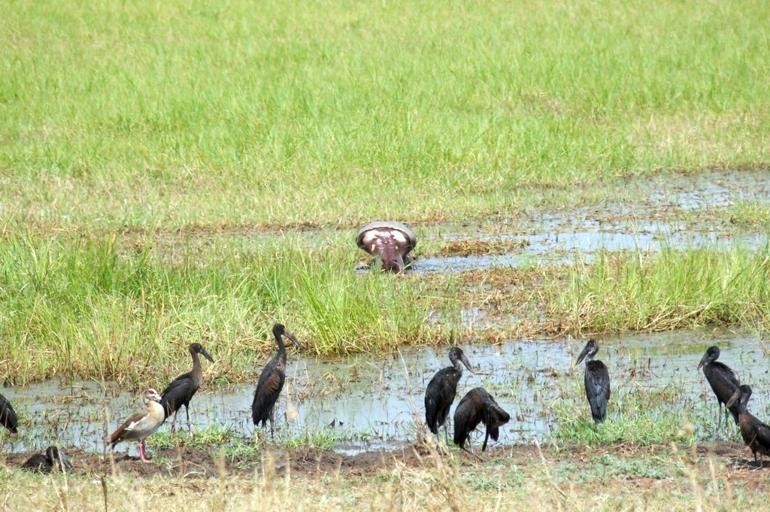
[
  {"xmin": 355, "ymin": 220, "xmax": 419, "ymax": 276},
  {"xmin": 451, "ymin": 385, "xmax": 511, "ymax": 464},
  {"xmin": 424, "ymin": 346, "xmax": 476, "ymax": 448},
  {"xmin": 696, "ymin": 345, "xmax": 741, "ymax": 430},
  {"xmin": 0, "ymin": 393, "xmax": 19, "ymax": 434},
  {"xmin": 104, "ymin": 388, "xmax": 166, "ymax": 464},
  {"xmin": 576, "ymin": 338, "xmax": 611, "ymax": 425},
  {"xmin": 156, "ymin": 342, "xmax": 216, "ymax": 434},
  {"xmin": 251, "ymin": 322, "xmax": 302, "ymax": 440},
  {"xmin": 19, "ymin": 445, "xmax": 74, "ymax": 475},
  {"xmin": 725, "ymin": 383, "xmax": 770, "ymax": 472}
]
